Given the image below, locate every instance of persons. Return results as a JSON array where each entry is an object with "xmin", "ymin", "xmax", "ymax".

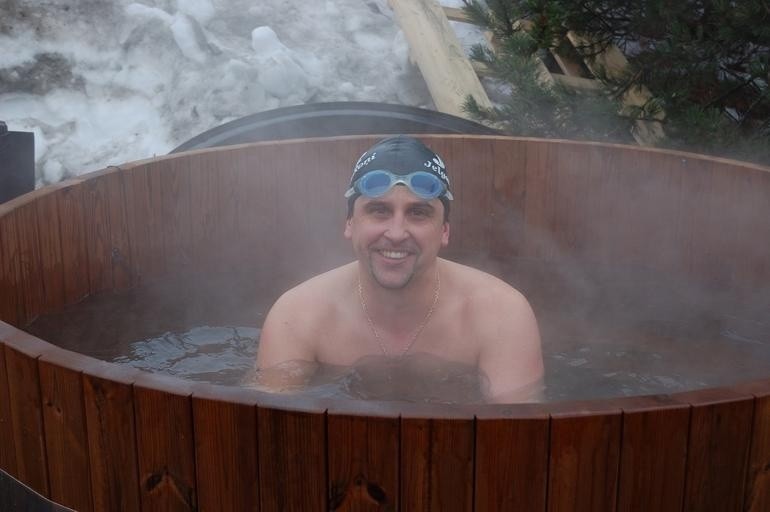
[{"xmin": 239, "ymin": 134, "xmax": 547, "ymax": 404}]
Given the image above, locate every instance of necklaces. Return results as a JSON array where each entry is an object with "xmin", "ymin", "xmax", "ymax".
[{"xmin": 357, "ymin": 268, "xmax": 441, "ymax": 386}]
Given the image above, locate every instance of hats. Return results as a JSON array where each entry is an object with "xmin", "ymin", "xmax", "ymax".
[{"xmin": 347, "ymin": 136, "xmax": 450, "ymax": 222}]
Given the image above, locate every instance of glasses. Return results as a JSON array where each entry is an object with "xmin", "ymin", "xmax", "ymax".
[{"xmin": 344, "ymin": 170, "xmax": 455, "ymax": 202}]
